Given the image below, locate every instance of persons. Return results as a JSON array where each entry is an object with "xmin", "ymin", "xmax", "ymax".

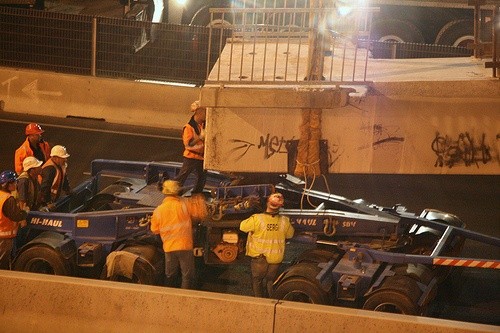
[
  {"xmin": 0, "ymin": 122, "xmax": 70, "ymax": 270},
  {"xmin": 240, "ymin": 193, "xmax": 295, "ymax": 299},
  {"xmin": 151, "ymin": 180, "xmax": 206, "ymax": 290},
  {"xmin": 176, "ymin": 100, "xmax": 206, "ymax": 195}
]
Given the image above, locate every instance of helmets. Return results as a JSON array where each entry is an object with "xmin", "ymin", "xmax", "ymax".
[
  {"xmin": 191, "ymin": 101, "xmax": 200, "ymax": 112},
  {"xmin": 25, "ymin": 123, "xmax": 45, "ymax": 135},
  {"xmin": 269, "ymin": 193, "xmax": 284, "ymax": 206},
  {"xmin": 162, "ymin": 180, "xmax": 179, "ymax": 195},
  {"xmin": 50, "ymin": 145, "xmax": 70, "ymax": 158},
  {"xmin": 23, "ymin": 157, "xmax": 43, "ymax": 172},
  {"xmin": 0, "ymin": 170, "xmax": 16, "ymax": 184}
]
